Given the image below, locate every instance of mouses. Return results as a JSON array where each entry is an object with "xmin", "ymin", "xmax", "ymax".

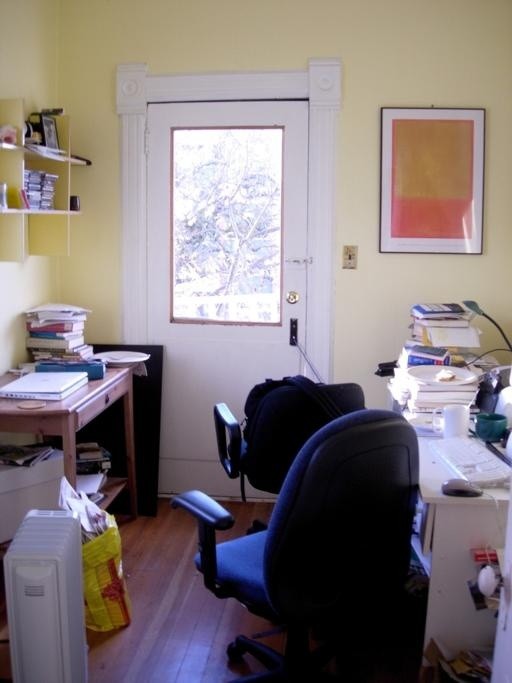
[{"xmin": 442, "ymin": 478, "xmax": 483, "ymax": 498}]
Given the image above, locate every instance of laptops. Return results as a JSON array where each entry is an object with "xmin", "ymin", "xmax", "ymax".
[{"xmin": 0, "ymin": 372, "xmax": 88, "ymax": 401}]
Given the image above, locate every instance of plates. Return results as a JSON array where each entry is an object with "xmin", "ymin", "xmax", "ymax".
[
  {"xmin": 406, "ymin": 364, "xmax": 476, "ymax": 386},
  {"xmin": 93, "ymin": 350, "xmax": 151, "ymax": 368}
]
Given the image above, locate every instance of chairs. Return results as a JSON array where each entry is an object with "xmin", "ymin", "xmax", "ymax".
[
  {"xmin": 167, "ymin": 408, "xmax": 419, "ymax": 683},
  {"xmin": 212, "ymin": 380, "xmax": 366, "ymax": 495}
]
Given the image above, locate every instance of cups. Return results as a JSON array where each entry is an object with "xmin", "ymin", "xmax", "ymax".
[
  {"xmin": 431, "ymin": 403, "xmax": 471, "ymax": 440},
  {"xmin": 474, "ymin": 412, "xmax": 507, "ymax": 441}
]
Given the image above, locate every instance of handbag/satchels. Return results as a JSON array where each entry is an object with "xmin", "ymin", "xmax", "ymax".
[
  {"xmin": 242, "ymin": 375, "xmax": 339, "ymax": 451},
  {"xmin": 82, "ymin": 512, "xmax": 131, "ymax": 633}
]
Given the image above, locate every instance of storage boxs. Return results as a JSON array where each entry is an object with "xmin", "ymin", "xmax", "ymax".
[{"xmin": 1, "ymin": 450, "xmax": 65, "ymax": 542}]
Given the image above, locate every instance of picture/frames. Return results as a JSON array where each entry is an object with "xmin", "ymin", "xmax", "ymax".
[{"xmin": 376, "ymin": 104, "xmax": 487, "ymax": 255}]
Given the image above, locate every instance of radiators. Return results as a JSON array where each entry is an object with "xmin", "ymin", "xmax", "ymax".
[{"xmin": 4, "ymin": 508, "xmax": 92, "ymax": 681}]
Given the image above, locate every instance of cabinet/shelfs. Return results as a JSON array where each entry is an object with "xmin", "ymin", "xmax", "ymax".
[{"xmin": 1, "ymin": 97, "xmax": 87, "ymax": 263}]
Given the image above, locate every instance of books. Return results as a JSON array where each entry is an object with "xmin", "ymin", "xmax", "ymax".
[
  {"xmin": 400, "ymin": 411, "xmax": 478, "ymax": 438},
  {"xmin": 17, "ymin": 301, "xmax": 96, "ymax": 370},
  {"xmin": 0, "ymin": 167, "xmax": 59, "ymax": 210},
  {"xmin": 424, "ymin": 637, "xmax": 493, "ymax": 682},
  {"xmin": 1, "ymin": 442, "xmax": 112, "ymax": 546},
  {"xmin": 384, "ymin": 299, "xmax": 499, "ymax": 415}
]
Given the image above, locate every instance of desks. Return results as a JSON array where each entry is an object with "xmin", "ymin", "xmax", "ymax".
[
  {"xmin": 1, "ymin": 362, "xmax": 141, "ymax": 530},
  {"xmin": 391, "ymin": 399, "xmax": 509, "ymax": 682}
]
